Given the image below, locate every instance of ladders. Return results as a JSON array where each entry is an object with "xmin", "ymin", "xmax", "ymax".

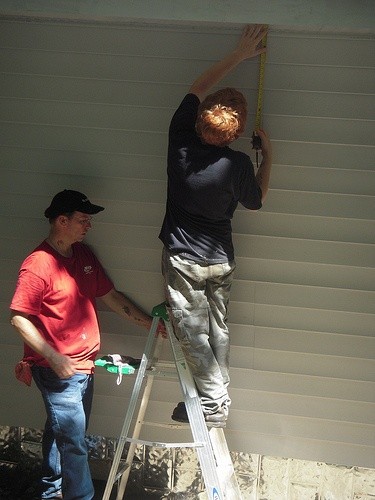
[{"xmin": 102, "ymin": 303, "xmax": 243, "ymax": 500}]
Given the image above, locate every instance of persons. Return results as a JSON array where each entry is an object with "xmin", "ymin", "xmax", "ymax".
[
  {"xmin": 8, "ymin": 188, "xmax": 167, "ymax": 500},
  {"xmin": 159, "ymin": 22, "xmax": 274, "ymax": 427}
]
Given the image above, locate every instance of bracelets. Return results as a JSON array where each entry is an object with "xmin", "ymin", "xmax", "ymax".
[{"xmin": 263, "ymin": 150, "xmax": 274, "ymax": 161}]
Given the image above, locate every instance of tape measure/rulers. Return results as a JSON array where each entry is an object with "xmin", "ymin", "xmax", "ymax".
[{"xmin": 252, "ymin": 23, "xmax": 268, "ymax": 168}]
